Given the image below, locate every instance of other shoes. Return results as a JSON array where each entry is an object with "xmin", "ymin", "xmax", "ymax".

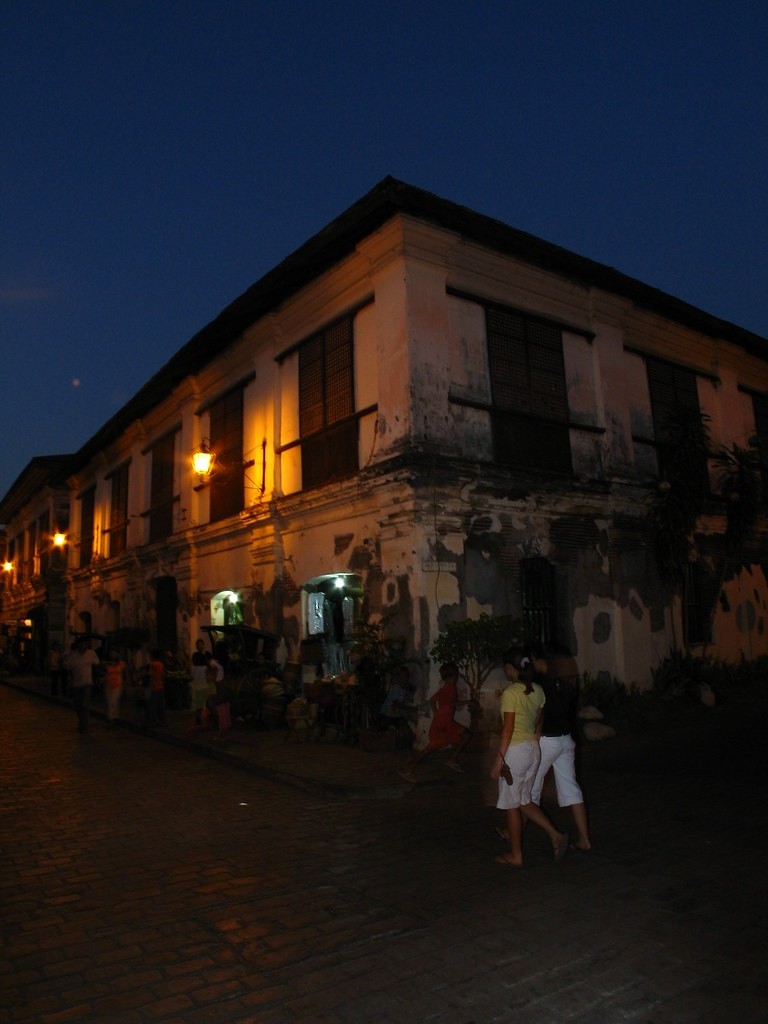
[
  {"xmin": 396, "ymin": 766, "xmax": 416, "ymax": 782},
  {"xmin": 442, "ymin": 760, "xmax": 462, "ymax": 774}
]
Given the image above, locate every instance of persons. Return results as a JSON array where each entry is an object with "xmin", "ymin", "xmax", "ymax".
[
  {"xmin": 489, "ymin": 647, "xmax": 568, "ymax": 866},
  {"xmin": 192, "ymin": 639, "xmax": 232, "ymax": 743},
  {"xmin": 400, "ymin": 662, "xmax": 484, "ymax": 782},
  {"xmin": 64, "ymin": 638, "xmax": 100, "ymax": 742},
  {"xmin": 50, "ymin": 635, "xmax": 191, "ymax": 726},
  {"xmin": 495, "ymin": 647, "xmax": 592, "ymax": 850}
]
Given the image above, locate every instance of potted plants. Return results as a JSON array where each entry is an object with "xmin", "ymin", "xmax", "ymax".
[{"xmin": 429, "ymin": 613, "xmax": 536, "ymax": 754}]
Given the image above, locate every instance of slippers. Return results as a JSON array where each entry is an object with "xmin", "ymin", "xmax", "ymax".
[
  {"xmin": 492, "ymin": 856, "xmax": 522, "ymax": 869},
  {"xmin": 493, "ymin": 826, "xmax": 511, "ymax": 844},
  {"xmin": 569, "ymin": 844, "xmax": 592, "ymax": 852},
  {"xmin": 553, "ymin": 835, "xmax": 569, "ymax": 862}
]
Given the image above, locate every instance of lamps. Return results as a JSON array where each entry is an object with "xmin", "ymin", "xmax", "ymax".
[{"xmin": 193, "ymin": 436, "xmax": 232, "ymax": 478}]
